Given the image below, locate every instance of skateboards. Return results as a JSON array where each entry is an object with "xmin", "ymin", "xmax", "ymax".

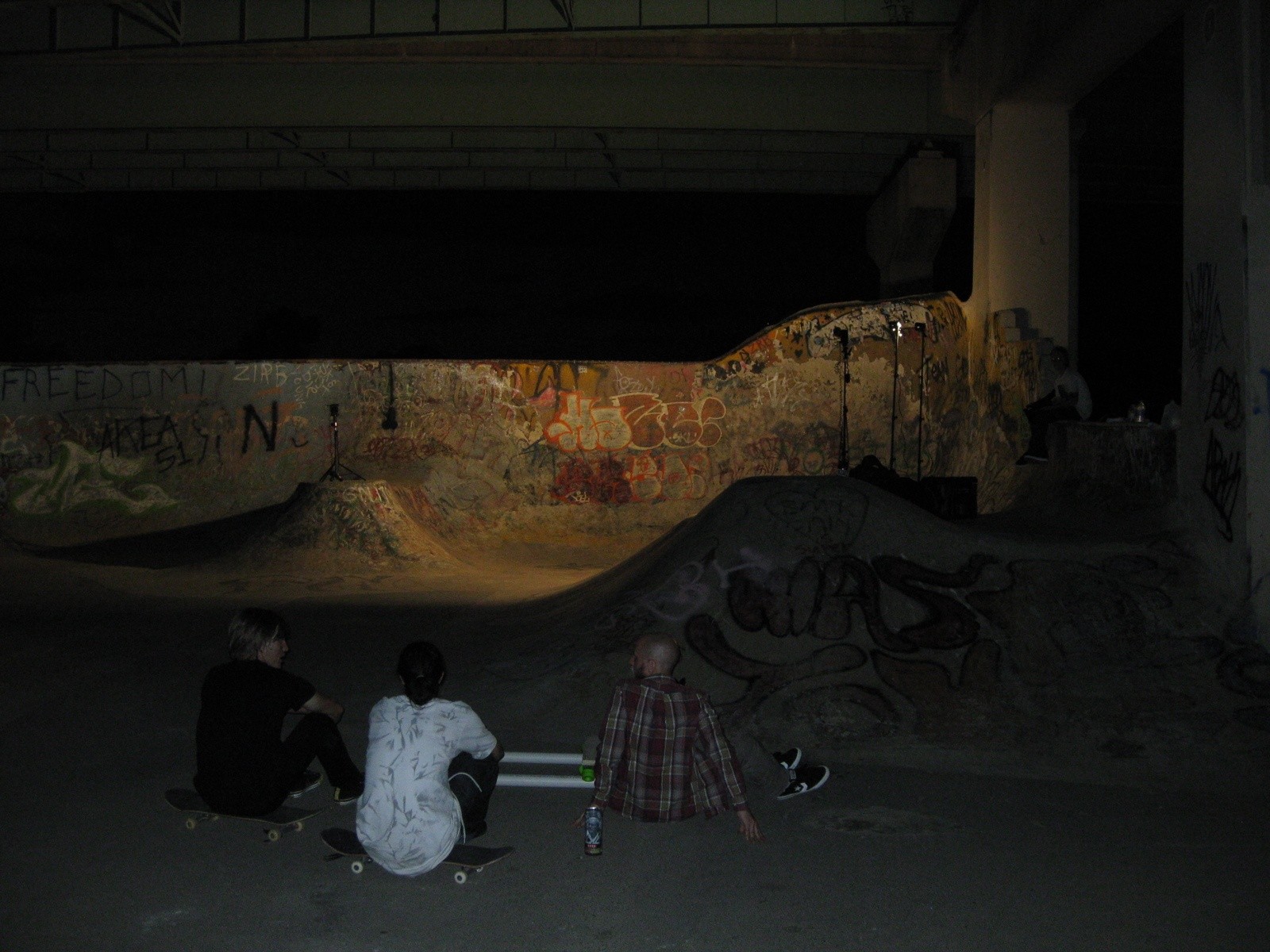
[
  {"xmin": 321, "ymin": 829, "xmax": 514, "ymax": 886},
  {"xmin": 164, "ymin": 787, "xmax": 322, "ymax": 841},
  {"xmin": 579, "ymin": 739, "xmax": 595, "ymax": 781}
]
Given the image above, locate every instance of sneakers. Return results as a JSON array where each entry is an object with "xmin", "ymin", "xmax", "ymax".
[
  {"xmin": 333, "ymin": 773, "xmax": 366, "ymax": 805},
  {"xmin": 288, "ymin": 772, "xmax": 324, "ymax": 798},
  {"xmin": 771, "ymin": 747, "xmax": 802, "ymax": 772},
  {"xmin": 778, "ymin": 764, "xmax": 830, "ymax": 801}
]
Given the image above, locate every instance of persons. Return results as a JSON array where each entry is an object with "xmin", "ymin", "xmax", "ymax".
[
  {"xmin": 1014, "ymin": 345, "xmax": 1091, "ymax": 467},
  {"xmin": 193, "ymin": 606, "xmax": 365, "ymax": 818},
  {"xmin": 357, "ymin": 644, "xmax": 504, "ymax": 880},
  {"xmin": 573, "ymin": 632, "xmax": 831, "ymax": 841}
]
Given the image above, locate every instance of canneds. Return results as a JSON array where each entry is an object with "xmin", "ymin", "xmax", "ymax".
[{"xmin": 584, "ymin": 806, "xmax": 603, "ymax": 856}]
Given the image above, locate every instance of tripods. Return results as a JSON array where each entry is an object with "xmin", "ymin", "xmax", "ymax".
[{"xmin": 319, "ymin": 422, "xmax": 367, "ymax": 482}]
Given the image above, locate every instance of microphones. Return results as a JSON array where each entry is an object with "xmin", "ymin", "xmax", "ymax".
[
  {"xmin": 329, "ymin": 403, "xmax": 339, "ymax": 429},
  {"xmin": 895, "ymin": 321, "xmax": 902, "ymax": 336},
  {"xmin": 833, "ymin": 327, "xmax": 849, "ymax": 346}
]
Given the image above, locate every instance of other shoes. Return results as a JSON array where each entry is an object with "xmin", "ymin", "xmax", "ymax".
[{"xmin": 460, "ymin": 821, "xmax": 489, "ymax": 846}]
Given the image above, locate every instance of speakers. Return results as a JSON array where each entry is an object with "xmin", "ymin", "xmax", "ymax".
[{"xmin": 921, "ymin": 475, "xmax": 979, "ymax": 517}]
